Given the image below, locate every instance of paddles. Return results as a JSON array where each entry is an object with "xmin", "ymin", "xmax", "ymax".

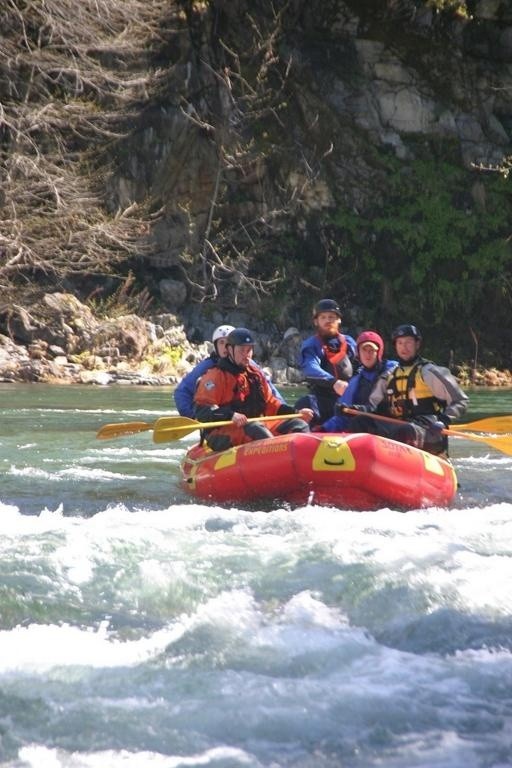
[
  {"xmin": 342, "ymin": 408, "xmax": 512, "ymax": 457},
  {"xmin": 96, "ymin": 413, "xmax": 306, "ymax": 444}
]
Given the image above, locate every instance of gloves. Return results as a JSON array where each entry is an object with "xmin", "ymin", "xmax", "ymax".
[
  {"xmin": 412, "ymin": 413, "xmax": 439, "ymax": 432},
  {"xmin": 332, "ymin": 401, "xmax": 350, "ymax": 417}
]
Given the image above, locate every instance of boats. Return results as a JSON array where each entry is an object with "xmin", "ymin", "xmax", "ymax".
[{"xmin": 180, "ymin": 429, "xmax": 456, "ymax": 513}]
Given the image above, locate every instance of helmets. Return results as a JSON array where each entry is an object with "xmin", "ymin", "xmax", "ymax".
[
  {"xmin": 211, "ymin": 324, "xmax": 237, "ymax": 344},
  {"xmin": 311, "ymin": 298, "xmax": 344, "ymax": 321},
  {"xmin": 355, "ymin": 330, "xmax": 385, "ymax": 364},
  {"xmin": 225, "ymin": 327, "xmax": 257, "ymax": 348},
  {"xmin": 391, "ymin": 323, "xmax": 424, "ymax": 346}
]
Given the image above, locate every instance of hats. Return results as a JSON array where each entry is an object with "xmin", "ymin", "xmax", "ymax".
[{"xmin": 359, "ymin": 340, "xmax": 380, "ymax": 351}]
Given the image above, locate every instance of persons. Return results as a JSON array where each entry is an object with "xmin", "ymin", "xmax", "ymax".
[{"xmin": 175, "ymin": 298, "xmax": 469, "ymax": 457}]
